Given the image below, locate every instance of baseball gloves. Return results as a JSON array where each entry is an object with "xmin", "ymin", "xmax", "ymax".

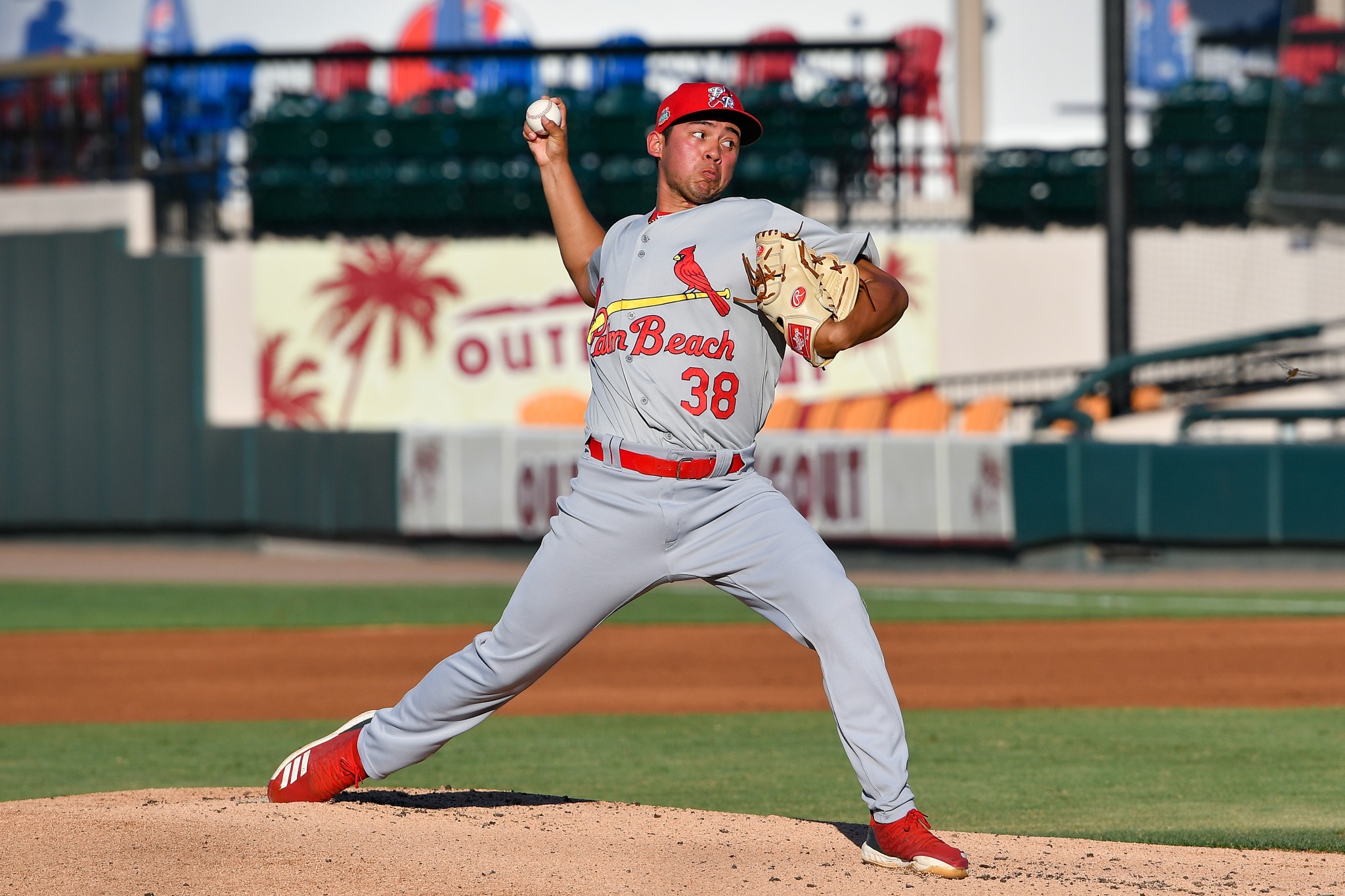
[{"xmin": 731, "ymin": 220, "xmax": 877, "ymax": 372}]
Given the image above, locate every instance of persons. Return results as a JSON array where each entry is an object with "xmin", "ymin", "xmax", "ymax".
[{"xmin": 267, "ymin": 84, "xmax": 971, "ymax": 881}]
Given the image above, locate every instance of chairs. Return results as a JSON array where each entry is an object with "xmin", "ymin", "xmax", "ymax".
[
  {"xmin": 515, "ymin": 386, "xmax": 1162, "ymax": 435},
  {"xmin": 23, "ymin": 0, "xmax": 1345, "ymax": 245}
]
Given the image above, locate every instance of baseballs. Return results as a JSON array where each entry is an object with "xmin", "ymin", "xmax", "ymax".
[{"xmin": 526, "ymin": 99, "xmax": 562, "ymax": 135}]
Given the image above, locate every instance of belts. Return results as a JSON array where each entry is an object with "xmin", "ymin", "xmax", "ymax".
[{"xmin": 589, "ymin": 437, "xmax": 744, "ymax": 481}]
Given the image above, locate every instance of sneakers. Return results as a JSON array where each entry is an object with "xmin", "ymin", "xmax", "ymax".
[
  {"xmin": 861, "ymin": 809, "xmax": 969, "ymax": 879},
  {"xmin": 267, "ymin": 709, "xmax": 377, "ymax": 803}
]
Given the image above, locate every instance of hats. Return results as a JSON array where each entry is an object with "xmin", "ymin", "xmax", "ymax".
[{"xmin": 655, "ymin": 82, "xmax": 762, "ymax": 147}]
